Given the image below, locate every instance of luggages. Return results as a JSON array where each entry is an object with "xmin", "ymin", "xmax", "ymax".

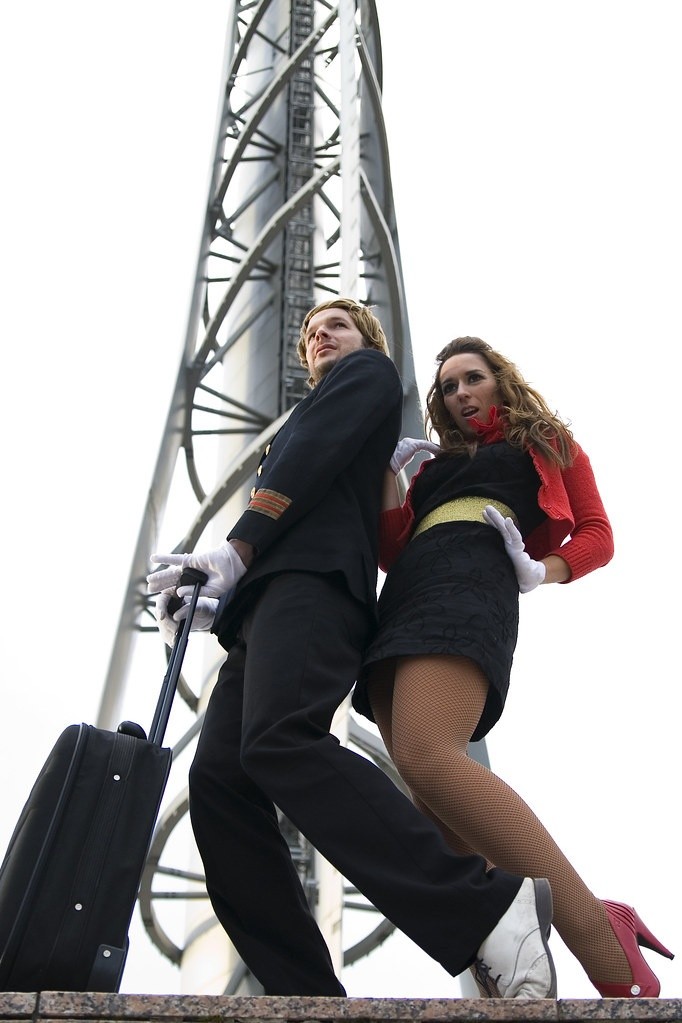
[{"xmin": 0, "ymin": 578, "xmax": 201, "ymax": 992}]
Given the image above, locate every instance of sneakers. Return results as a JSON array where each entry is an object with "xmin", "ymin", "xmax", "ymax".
[{"xmin": 469, "ymin": 876, "xmax": 558, "ymax": 999}]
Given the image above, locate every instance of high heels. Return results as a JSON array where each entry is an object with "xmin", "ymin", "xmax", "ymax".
[{"xmin": 590, "ymin": 899, "xmax": 674, "ymax": 998}]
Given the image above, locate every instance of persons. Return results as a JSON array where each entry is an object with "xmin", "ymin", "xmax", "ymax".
[
  {"xmin": 350, "ymin": 337, "xmax": 674, "ymax": 1001},
  {"xmin": 146, "ymin": 299, "xmax": 556, "ymax": 1001}
]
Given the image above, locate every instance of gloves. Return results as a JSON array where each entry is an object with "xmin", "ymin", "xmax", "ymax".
[
  {"xmin": 481, "ymin": 503, "xmax": 547, "ymax": 594},
  {"xmin": 155, "ymin": 587, "xmax": 219, "ymax": 650},
  {"xmin": 147, "ymin": 541, "xmax": 245, "ymax": 598},
  {"xmin": 389, "ymin": 437, "xmax": 442, "ymax": 474}
]
